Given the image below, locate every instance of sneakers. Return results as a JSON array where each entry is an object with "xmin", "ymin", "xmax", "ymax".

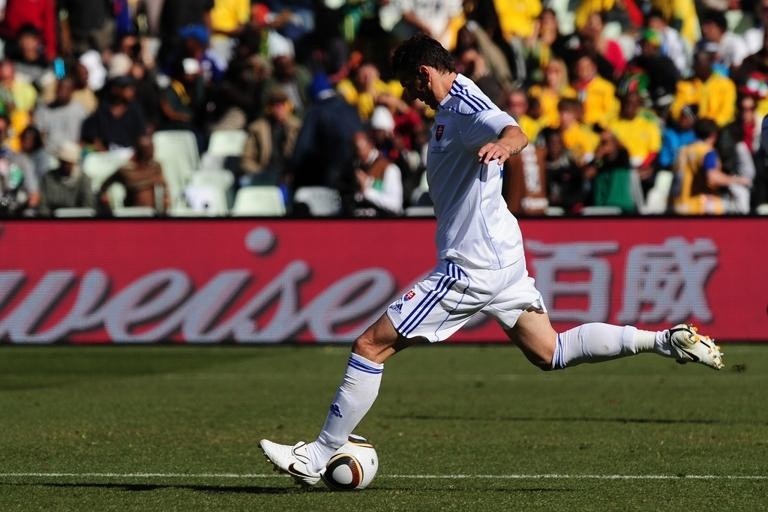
[
  {"xmin": 258, "ymin": 439, "xmax": 327, "ymax": 492},
  {"xmin": 663, "ymin": 324, "xmax": 725, "ymax": 370}
]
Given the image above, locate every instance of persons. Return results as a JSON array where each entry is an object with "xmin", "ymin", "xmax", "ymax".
[
  {"xmin": 0, "ymin": 1, "xmax": 767, "ymax": 216},
  {"xmin": 257, "ymin": 31, "xmax": 724, "ymax": 491}
]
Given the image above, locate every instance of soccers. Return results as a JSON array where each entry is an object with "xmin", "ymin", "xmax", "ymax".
[{"xmin": 321, "ymin": 435, "xmax": 378, "ymax": 491}]
